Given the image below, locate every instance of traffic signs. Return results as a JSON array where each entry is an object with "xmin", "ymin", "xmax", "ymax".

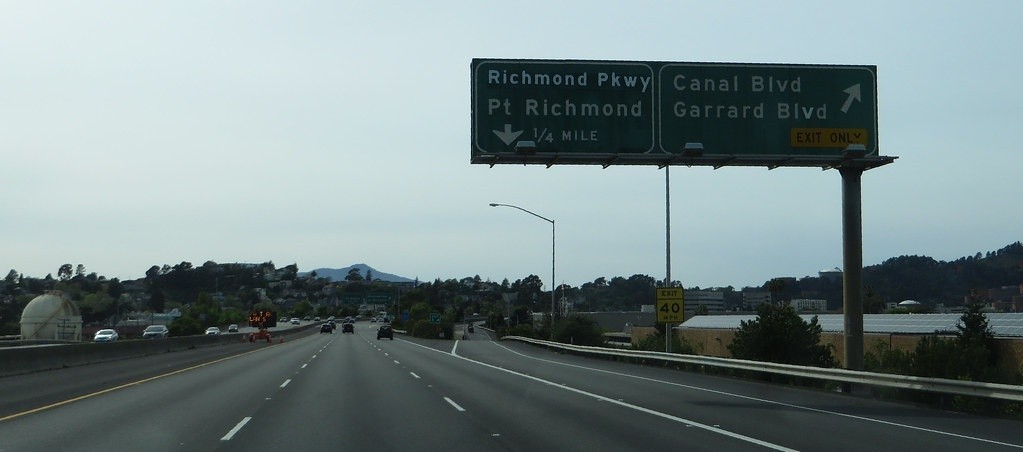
[{"xmin": 470, "ymin": 57, "xmax": 880, "ymax": 166}]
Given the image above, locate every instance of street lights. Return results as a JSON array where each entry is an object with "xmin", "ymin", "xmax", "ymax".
[{"xmin": 491, "ymin": 202, "xmax": 559, "ymax": 353}]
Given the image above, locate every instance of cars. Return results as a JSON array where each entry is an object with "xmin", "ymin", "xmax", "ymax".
[
  {"xmin": 228, "ymin": 324, "xmax": 237, "ymax": 332},
  {"xmin": 142, "ymin": 324, "xmax": 169, "ymax": 339},
  {"xmin": 91, "ymin": 329, "xmax": 118, "ymax": 343},
  {"xmin": 376, "ymin": 326, "xmax": 394, "ymax": 341},
  {"xmin": 205, "ymin": 327, "xmax": 221, "ymax": 336},
  {"xmin": 278, "ymin": 315, "xmax": 391, "ymax": 334}
]
